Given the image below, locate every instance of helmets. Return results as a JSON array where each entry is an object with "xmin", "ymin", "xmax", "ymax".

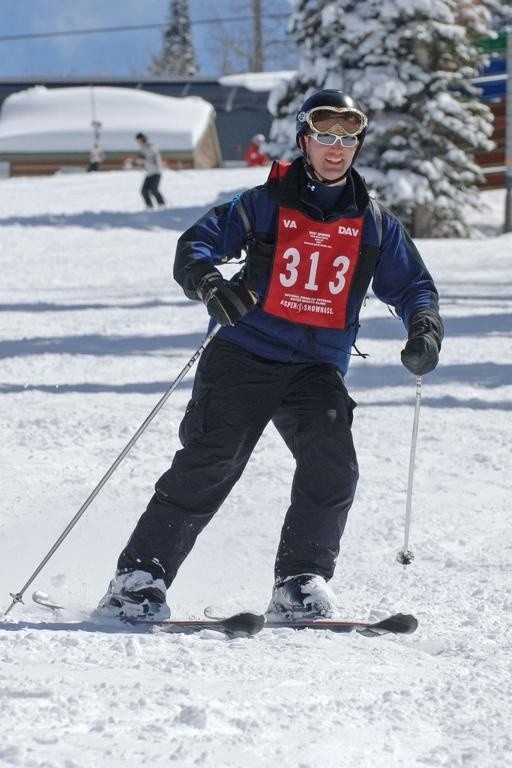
[{"xmin": 294, "ymin": 88, "xmax": 367, "ymax": 148}]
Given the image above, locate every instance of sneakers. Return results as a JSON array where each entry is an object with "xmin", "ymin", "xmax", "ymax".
[
  {"xmin": 94, "ymin": 567, "xmax": 171, "ymax": 621},
  {"xmin": 267, "ymin": 574, "xmax": 333, "ymax": 619}
]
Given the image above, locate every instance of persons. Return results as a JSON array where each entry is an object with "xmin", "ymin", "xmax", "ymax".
[
  {"xmin": 135, "ymin": 132, "xmax": 166, "ymax": 208},
  {"xmin": 103, "ymin": 89, "xmax": 442, "ymax": 620},
  {"xmin": 243, "ymin": 134, "xmax": 270, "ymax": 167}
]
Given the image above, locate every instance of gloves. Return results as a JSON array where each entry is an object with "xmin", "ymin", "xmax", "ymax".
[
  {"xmin": 400, "ymin": 306, "xmax": 445, "ymax": 375},
  {"xmin": 197, "ymin": 272, "xmax": 257, "ymax": 327}
]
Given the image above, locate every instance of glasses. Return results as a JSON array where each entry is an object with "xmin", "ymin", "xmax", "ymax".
[
  {"xmin": 299, "ymin": 107, "xmax": 367, "ymax": 138},
  {"xmin": 306, "ymin": 132, "xmax": 360, "ymax": 149}
]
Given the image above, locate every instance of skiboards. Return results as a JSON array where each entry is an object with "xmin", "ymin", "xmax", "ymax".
[{"xmin": 33, "ymin": 591, "xmax": 418, "ymax": 637}]
